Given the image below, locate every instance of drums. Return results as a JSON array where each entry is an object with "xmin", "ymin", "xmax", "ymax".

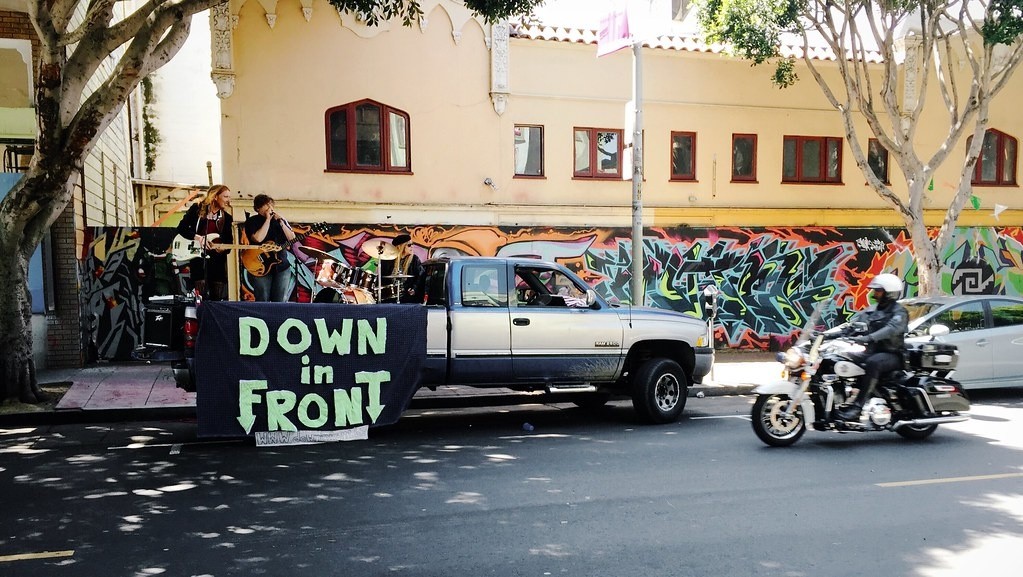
[
  {"xmin": 313, "ymin": 286, "xmax": 374, "ymax": 305},
  {"xmin": 379, "ymin": 283, "xmax": 408, "ymax": 299},
  {"xmin": 331, "ymin": 261, "xmax": 351, "ymax": 283},
  {"xmin": 352, "ymin": 267, "xmax": 377, "ymax": 290}
]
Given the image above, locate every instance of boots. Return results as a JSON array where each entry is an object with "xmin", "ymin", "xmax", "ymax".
[{"xmin": 835, "ymin": 377, "xmax": 878, "ymax": 421}]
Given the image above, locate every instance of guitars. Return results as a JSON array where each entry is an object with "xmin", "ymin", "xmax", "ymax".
[
  {"xmin": 240, "ymin": 221, "xmax": 331, "ymax": 277},
  {"xmin": 172, "ymin": 234, "xmax": 280, "ymax": 261}
]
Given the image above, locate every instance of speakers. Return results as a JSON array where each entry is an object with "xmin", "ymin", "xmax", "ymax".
[{"xmin": 144, "ymin": 308, "xmax": 186, "ymax": 349}]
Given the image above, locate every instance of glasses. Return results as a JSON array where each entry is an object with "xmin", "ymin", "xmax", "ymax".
[{"xmin": 407, "ymin": 243, "xmax": 413, "ymax": 247}]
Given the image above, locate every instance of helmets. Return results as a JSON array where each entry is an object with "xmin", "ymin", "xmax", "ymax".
[{"xmin": 865, "ymin": 274, "xmax": 904, "ymax": 302}]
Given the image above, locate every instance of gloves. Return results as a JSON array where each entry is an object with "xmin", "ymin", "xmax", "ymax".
[{"xmin": 849, "ymin": 335, "xmax": 867, "ymax": 345}]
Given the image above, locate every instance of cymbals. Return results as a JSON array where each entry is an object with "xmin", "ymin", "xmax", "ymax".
[
  {"xmin": 361, "ymin": 240, "xmax": 396, "ymax": 260},
  {"xmin": 298, "ymin": 245, "xmax": 342, "ymax": 263},
  {"xmin": 382, "ymin": 274, "xmax": 414, "ymax": 278}
]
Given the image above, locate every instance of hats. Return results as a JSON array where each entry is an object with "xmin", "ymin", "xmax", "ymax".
[{"xmin": 392, "ymin": 234, "xmax": 411, "ymax": 246}]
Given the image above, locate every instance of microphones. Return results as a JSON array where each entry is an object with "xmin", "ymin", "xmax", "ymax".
[
  {"xmin": 271, "ymin": 213, "xmax": 280, "ymax": 227},
  {"xmin": 206, "ymin": 204, "xmax": 211, "ymax": 216}
]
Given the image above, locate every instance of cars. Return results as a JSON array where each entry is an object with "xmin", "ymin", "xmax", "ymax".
[{"xmin": 799, "ymin": 296, "xmax": 1023, "ymax": 394}]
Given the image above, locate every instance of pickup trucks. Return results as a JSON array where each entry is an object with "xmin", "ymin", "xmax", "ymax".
[{"xmin": 170, "ymin": 256, "xmax": 715, "ymax": 424}]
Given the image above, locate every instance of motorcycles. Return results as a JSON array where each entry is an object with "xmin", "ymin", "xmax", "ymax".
[{"xmin": 750, "ymin": 298, "xmax": 970, "ymax": 448}]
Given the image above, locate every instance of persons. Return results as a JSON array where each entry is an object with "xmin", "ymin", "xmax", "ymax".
[
  {"xmin": 245, "ymin": 194, "xmax": 296, "ymax": 302},
  {"xmin": 392, "ymin": 234, "xmax": 423, "ymax": 303},
  {"xmin": 824, "ymin": 274, "xmax": 910, "ymax": 422},
  {"xmin": 316, "ymin": 258, "xmax": 337, "ymax": 295},
  {"xmin": 176, "ymin": 185, "xmax": 234, "ymax": 300}
]
